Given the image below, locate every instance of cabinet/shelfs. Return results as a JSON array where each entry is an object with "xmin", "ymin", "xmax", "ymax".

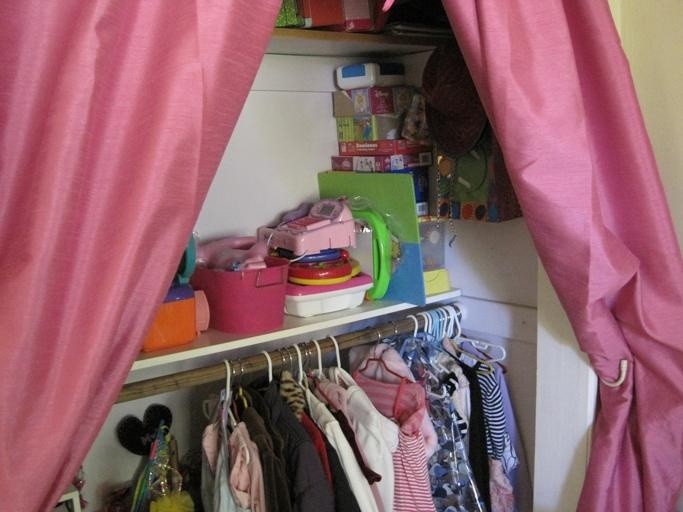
[{"xmin": 59, "ymin": 28, "xmax": 460, "ymax": 377}]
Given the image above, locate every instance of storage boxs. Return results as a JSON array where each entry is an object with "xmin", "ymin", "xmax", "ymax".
[{"xmin": 417, "ymin": 215, "xmax": 446, "ymax": 270}]
